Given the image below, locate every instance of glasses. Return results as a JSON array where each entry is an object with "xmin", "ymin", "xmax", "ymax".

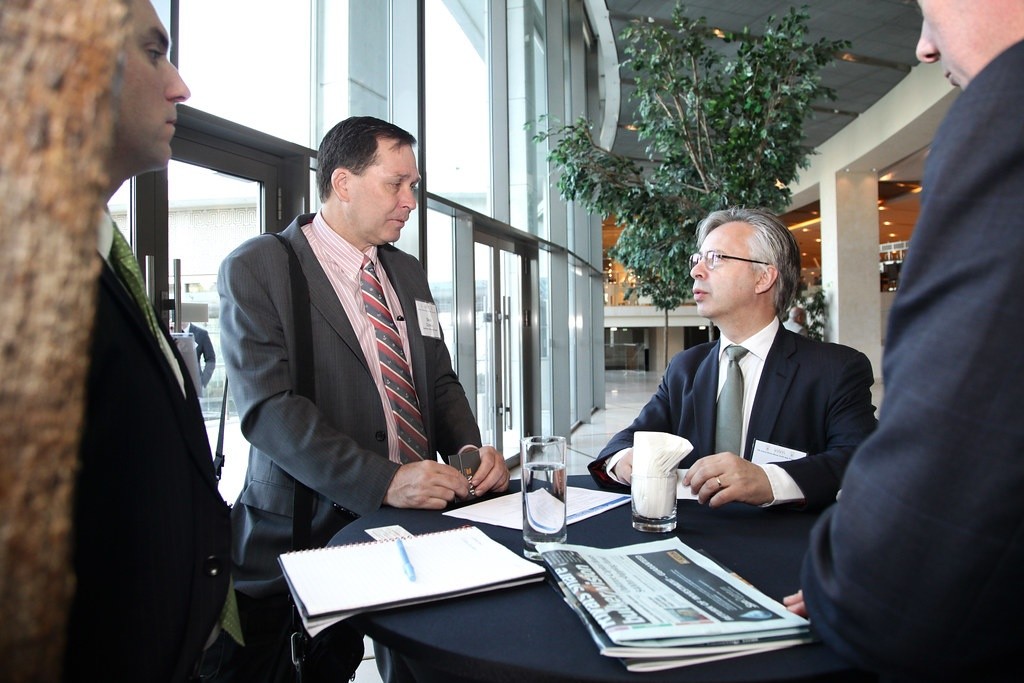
[{"xmin": 688, "ymin": 252, "xmax": 770, "ymax": 270}]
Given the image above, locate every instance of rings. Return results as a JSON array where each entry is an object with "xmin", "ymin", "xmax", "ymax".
[{"xmin": 716, "ymin": 477, "xmax": 722, "ymax": 491}]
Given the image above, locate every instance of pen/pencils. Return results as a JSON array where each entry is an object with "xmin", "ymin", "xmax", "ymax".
[
  {"xmin": 397, "ymin": 314, "xmax": 405, "ymax": 322},
  {"xmin": 395, "ymin": 536, "xmax": 416, "ymax": 583}
]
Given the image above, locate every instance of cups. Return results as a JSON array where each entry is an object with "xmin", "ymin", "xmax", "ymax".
[
  {"xmin": 630, "ymin": 473, "xmax": 677, "ymax": 532},
  {"xmin": 521, "ymin": 436, "xmax": 567, "ymax": 561}
]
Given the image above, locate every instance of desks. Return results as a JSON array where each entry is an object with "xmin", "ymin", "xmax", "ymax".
[
  {"xmin": 326, "ymin": 474, "xmax": 841, "ymax": 683},
  {"xmin": 880, "ymin": 260, "xmax": 903, "ymax": 292}
]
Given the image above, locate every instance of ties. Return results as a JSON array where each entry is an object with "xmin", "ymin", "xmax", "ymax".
[
  {"xmin": 361, "ymin": 255, "xmax": 429, "ymax": 464},
  {"xmin": 713, "ymin": 346, "xmax": 749, "ymax": 457},
  {"xmin": 111, "ymin": 221, "xmax": 172, "ymax": 367}
]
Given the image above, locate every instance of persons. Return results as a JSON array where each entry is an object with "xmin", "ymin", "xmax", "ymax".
[
  {"xmin": 216, "ymin": 116, "xmax": 510, "ymax": 683},
  {"xmin": 783, "ymin": 0, "xmax": 1024, "ymax": 683},
  {"xmin": 49, "ymin": 0, "xmax": 232, "ymax": 683},
  {"xmin": 782, "ymin": 307, "xmax": 807, "ymax": 337},
  {"xmin": 586, "ymin": 208, "xmax": 878, "ymax": 508},
  {"xmin": 169, "ymin": 319, "xmax": 216, "ymax": 388}
]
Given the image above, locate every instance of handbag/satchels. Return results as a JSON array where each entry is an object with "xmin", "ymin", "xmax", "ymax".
[{"xmin": 191, "ymin": 623, "xmax": 364, "ymax": 683}]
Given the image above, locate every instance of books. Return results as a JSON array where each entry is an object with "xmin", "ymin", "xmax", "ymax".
[
  {"xmin": 277, "ymin": 525, "xmax": 545, "ymax": 637},
  {"xmin": 537, "ymin": 537, "xmax": 813, "ymax": 672}
]
